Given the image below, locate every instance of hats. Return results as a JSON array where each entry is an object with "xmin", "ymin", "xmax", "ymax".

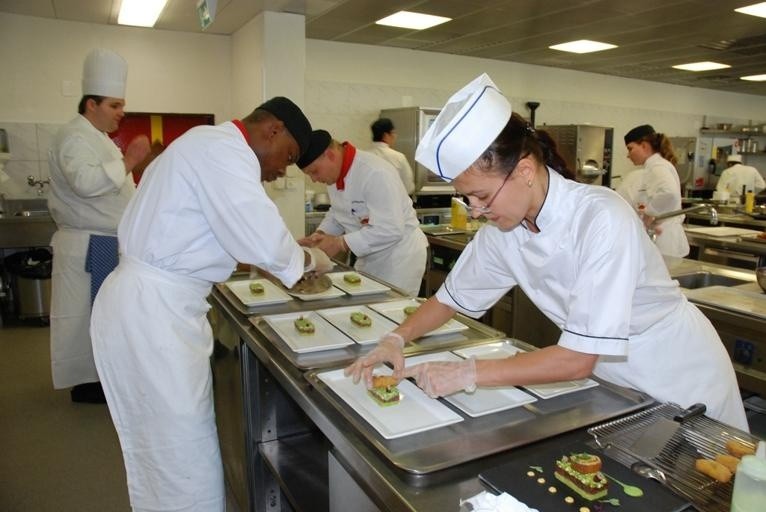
[
  {"xmin": 726, "ymin": 154, "xmax": 743, "ymax": 163},
  {"xmin": 624, "ymin": 125, "xmax": 655, "ymax": 145},
  {"xmin": 81, "ymin": 48, "xmax": 127, "ymax": 99},
  {"xmin": 371, "ymin": 118, "xmax": 393, "ymax": 136},
  {"xmin": 415, "ymin": 72, "xmax": 512, "ymax": 183},
  {"xmin": 255, "ymin": 97, "xmax": 312, "ymax": 165},
  {"xmin": 296, "ymin": 129, "xmax": 332, "ymax": 169}
]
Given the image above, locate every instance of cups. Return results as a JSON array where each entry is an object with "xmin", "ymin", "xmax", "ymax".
[{"xmin": 730, "ymin": 454, "xmax": 766, "ymax": 512}]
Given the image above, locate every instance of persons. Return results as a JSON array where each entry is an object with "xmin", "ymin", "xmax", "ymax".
[
  {"xmin": 366, "ymin": 118, "xmax": 416, "ymax": 200},
  {"xmin": 623, "ymin": 125, "xmax": 690, "ymax": 259},
  {"xmin": 294, "ymin": 130, "xmax": 429, "ymax": 299},
  {"xmin": 89, "ymin": 95, "xmax": 333, "ymax": 512},
  {"xmin": 715, "ymin": 155, "xmax": 766, "ymax": 195},
  {"xmin": 48, "ymin": 95, "xmax": 153, "ymax": 406},
  {"xmin": 343, "ymin": 111, "xmax": 751, "ymax": 457}
]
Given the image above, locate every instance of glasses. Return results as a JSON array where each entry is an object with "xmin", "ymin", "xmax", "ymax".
[{"xmin": 451, "ymin": 151, "xmax": 532, "ymax": 213}]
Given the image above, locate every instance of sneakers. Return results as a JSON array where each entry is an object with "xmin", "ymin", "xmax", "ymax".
[{"xmin": 70, "ymin": 381, "xmax": 107, "ymax": 404}]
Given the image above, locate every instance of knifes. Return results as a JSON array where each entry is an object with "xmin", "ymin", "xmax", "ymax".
[{"xmin": 628, "ymin": 403, "xmax": 706, "ymax": 459}]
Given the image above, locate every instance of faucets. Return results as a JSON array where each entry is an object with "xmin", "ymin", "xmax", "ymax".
[
  {"xmin": 36, "ymin": 175, "xmax": 44, "ymax": 194},
  {"xmin": 647, "ymin": 204, "xmax": 718, "ymax": 243}
]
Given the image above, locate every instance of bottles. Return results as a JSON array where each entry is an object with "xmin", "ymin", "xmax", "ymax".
[
  {"xmin": 737, "ymin": 138, "xmax": 759, "ymax": 153},
  {"xmin": 451, "ymin": 197, "xmax": 480, "ymax": 231},
  {"xmin": 744, "ymin": 190, "xmax": 755, "ymax": 214}
]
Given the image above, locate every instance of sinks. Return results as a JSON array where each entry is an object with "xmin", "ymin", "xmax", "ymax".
[
  {"xmin": 11, "ymin": 210, "xmax": 51, "ymax": 218},
  {"xmin": 670, "ymin": 271, "xmax": 754, "ymax": 290}
]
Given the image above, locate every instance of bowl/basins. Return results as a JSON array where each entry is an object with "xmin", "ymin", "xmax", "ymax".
[
  {"xmin": 755, "ymin": 267, "xmax": 766, "ymax": 294},
  {"xmin": 716, "ymin": 123, "xmax": 733, "ymax": 130}
]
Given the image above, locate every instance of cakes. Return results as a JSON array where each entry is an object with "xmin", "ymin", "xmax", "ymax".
[
  {"xmin": 344, "ymin": 274, "xmax": 361, "ymax": 285},
  {"xmin": 402, "ymin": 305, "xmax": 419, "ymax": 320},
  {"xmin": 367, "ymin": 375, "xmax": 400, "ymax": 407},
  {"xmin": 351, "ymin": 313, "xmax": 371, "ymax": 328},
  {"xmin": 555, "ymin": 453, "xmax": 607, "ymax": 501},
  {"xmin": 250, "ymin": 284, "xmax": 263, "ymax": 295},
  {"xmin": 293, "ymin": 316, "xmax": 314, "ymax": 336}
]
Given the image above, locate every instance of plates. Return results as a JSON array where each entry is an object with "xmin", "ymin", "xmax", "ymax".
[
  {"xmin": 323, "ymin": 270, "xmax": 391, "ymax": 297},
  {"xmin": 477, "ymin": 441, "xmax": 693, "ymax": 512},
  {"xmin": 261, "ymin": 296, "xmax": 470, "ymax": 354},
  {"xmin": 224, "ymin": 277, "xmax": 294, "ymax": 308},
  {"xmin": 288, "ymin": 285, "xmax": 346, "ymax": 302},
  {"xmin": 315, "ymin": 341, "xmax": 601, "ymax": 440}
]
